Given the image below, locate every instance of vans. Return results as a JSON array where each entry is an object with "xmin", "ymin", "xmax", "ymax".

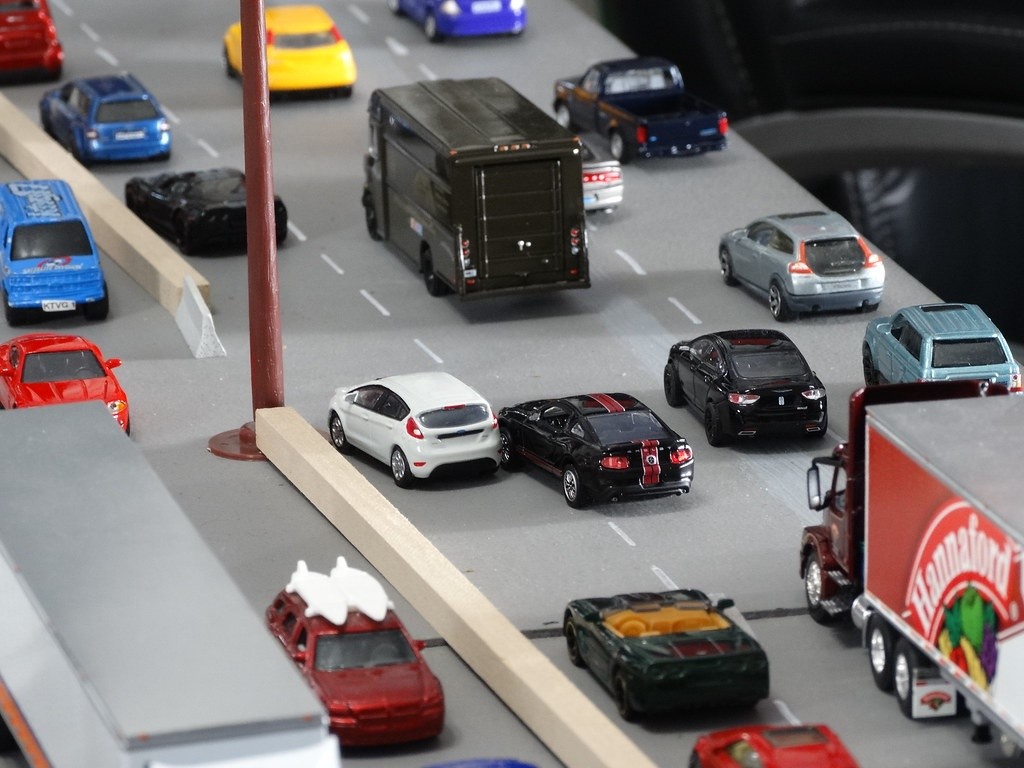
[{"xmin": 1, "ymin": 179, "xmax": 110, "ymax": 325}]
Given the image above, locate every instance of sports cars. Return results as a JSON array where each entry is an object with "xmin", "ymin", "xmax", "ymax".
[
  {"xmin": 123, "ymin": 164, "xmax": 288, "ymax": 254},
  {"xmin": 499, "ymin": 396, "xmax": 696, "ymax": 511},
  {"xmin": 563, "ymin": 589, "xmax": 772, "ymax": 726}
]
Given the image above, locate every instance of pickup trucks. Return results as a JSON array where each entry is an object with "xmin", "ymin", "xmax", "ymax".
[{"xmin": 552, "ymin": 55, "xmax": 732, "ymax": 164}]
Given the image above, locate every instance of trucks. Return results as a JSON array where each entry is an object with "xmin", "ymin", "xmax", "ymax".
[{"xmin": 359, "ymin": 74, "xmax": 593, "ymax": 299}]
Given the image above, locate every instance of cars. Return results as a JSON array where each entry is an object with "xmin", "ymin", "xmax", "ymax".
[
  {"xmin": 393, "ymin": 0, "xmax": 530, "ymax": 41},
  {"xmin": 716, "ymin": 210, "xmax": 885, "ymax": 324},
  {"xmin": 0, "ymin": 334, "xmax": 132, "ymax": 436},
  {"xmin": 224, "ymin": 4, "xmax": 359, "ymax": 101},
  {"xmin": 0, "ymin": 0, "xmax": 67, "ymax": 84},
  {"xmin": 270, "ymin": 557, "xmax": 449, "ymax": 753},
  {"xmin": 578, "ymin": 140, "xmax": 623, "ymax": 217},
  {"xmin": 687, "ymin": 721, "xmax": 864, "ymax": 768},
  {"xmin": 663, "ymin": 326, "xmax": 829, "ymax": 448}
]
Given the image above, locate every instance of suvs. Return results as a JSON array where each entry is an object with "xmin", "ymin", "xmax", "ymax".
[
  {"xmin": 37, "ymin": 69, "xmax": 174, "ymax": 162},
  {"xmin": 324, "ymin": 369, "xmax": 503, "ymax": 487},
  {"xmin": 859, "ymin": 301, "xmax": 1023, "ymax": 398}
]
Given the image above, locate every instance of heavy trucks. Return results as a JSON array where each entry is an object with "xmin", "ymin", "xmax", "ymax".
[{"xmin": 798, "ymin": 377, "xmax": 1024, "ymax": 758}]
